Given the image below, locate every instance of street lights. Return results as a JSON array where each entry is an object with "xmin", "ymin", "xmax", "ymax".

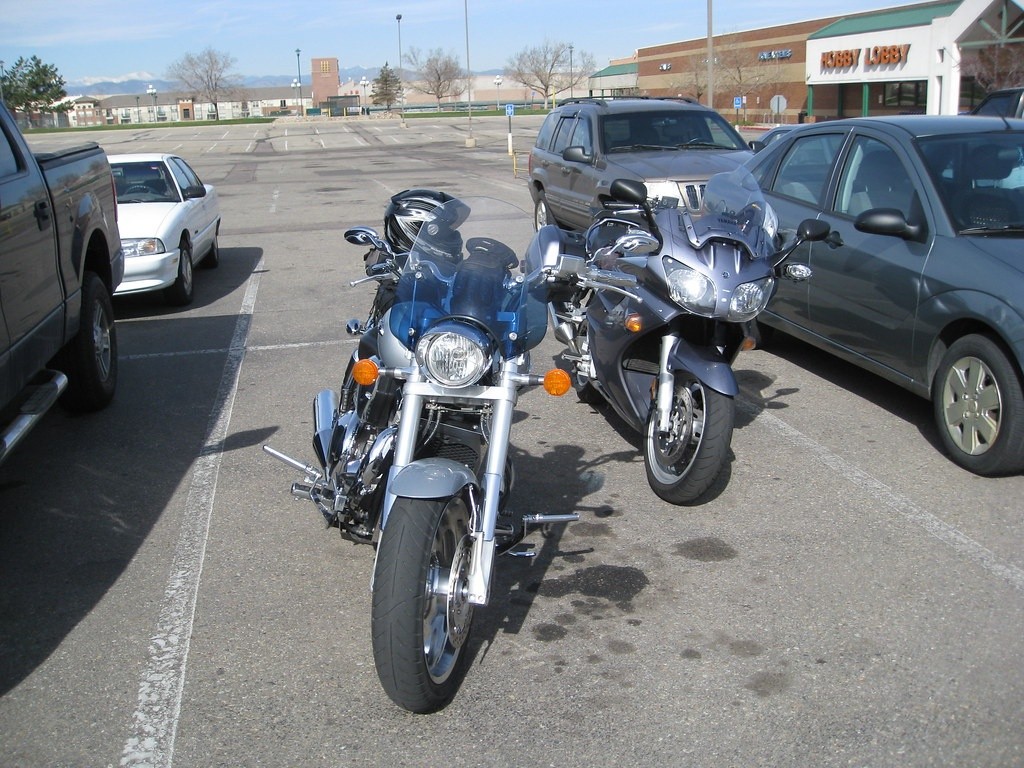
[
  {"xmin": 493, "ymin": 75, "xmax": 503, "ymax": 111},
  {"xmin": 360, "ymin": 76, "xmax": 370, "ymax": 115},
  {"xmin": 291, "ymin": 79, "xmax": 301, "ymax": 118},
  {"xmin": 568, "ymin": 45, "xmax": 574, "ymax": 99},
  {"xmin": 294, "ymin": 48, "xmax": 304, "ymax": 117},
  {"xmin": 147, "ymin": 84, "xmax": 157, "ymax": 122}
]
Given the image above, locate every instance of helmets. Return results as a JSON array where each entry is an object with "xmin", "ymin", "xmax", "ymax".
[{"xmin": 383, "ymin": 189, "xmax": 471, "ymax": 279}]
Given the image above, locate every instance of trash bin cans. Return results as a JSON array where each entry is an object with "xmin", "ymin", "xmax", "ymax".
[
  {"xmin": 798, "ymin": 110, "xmax": 808, "ymax": 124},
  {"xmin": 362, "ymin": 107, "xmax": 370, "ymax": 115}
]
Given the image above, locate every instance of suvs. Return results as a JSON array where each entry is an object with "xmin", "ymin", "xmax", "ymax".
[{"xmin": 527, "ymin": 93, "xmax": 764, "ymax": 239}]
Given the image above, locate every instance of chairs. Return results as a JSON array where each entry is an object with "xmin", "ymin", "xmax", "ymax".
[
  {"xmin": 662, "ymin": 124, "xmax": 688, "ymax": 147},
  {"xmin": 143, "ymin": 179, "xmax": 167, "ymax": 196},
  {"xmin": 848, "ymin": 151, "xmax": 920, "ymax": 227}
]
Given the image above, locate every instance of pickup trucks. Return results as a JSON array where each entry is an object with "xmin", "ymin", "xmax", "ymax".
[{"xmin": 1, "ymin": 93, "xmax": 124, "ymax": 469}]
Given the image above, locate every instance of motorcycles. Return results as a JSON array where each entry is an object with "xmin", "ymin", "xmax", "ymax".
[
  {"xmin": 262, "ymin": 195, "xmax": 661, "ymax": 714},
  {"xmin": 524, "ymin": 168, "xmax": 843, "ymax": 506}
]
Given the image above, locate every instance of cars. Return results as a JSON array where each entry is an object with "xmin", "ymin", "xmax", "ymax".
[
  {"xmin": 700, "ymin": 116, "xmax": 1024, "ymax": 478},
  {"xmin": 113, "ymin": 153, "xmax": 222, "ymax": 308}
]
